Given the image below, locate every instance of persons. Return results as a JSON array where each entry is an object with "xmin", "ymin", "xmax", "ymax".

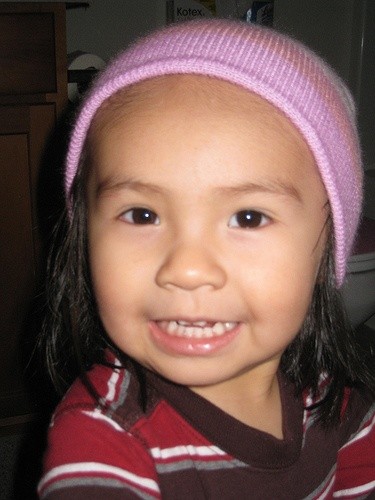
[{"xmin": 35, "ymin": 20, "xmax": 375, "ymax": 500}]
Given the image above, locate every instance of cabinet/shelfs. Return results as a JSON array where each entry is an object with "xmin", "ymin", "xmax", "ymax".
[{"xmin": 0, "ymin": 0, "xmax": 75, "ymax": 431}]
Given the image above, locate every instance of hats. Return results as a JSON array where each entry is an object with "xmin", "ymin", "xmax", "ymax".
[{"xmin": 64, "ymin": 17, "xmax": 365, "ymax": 287}]
